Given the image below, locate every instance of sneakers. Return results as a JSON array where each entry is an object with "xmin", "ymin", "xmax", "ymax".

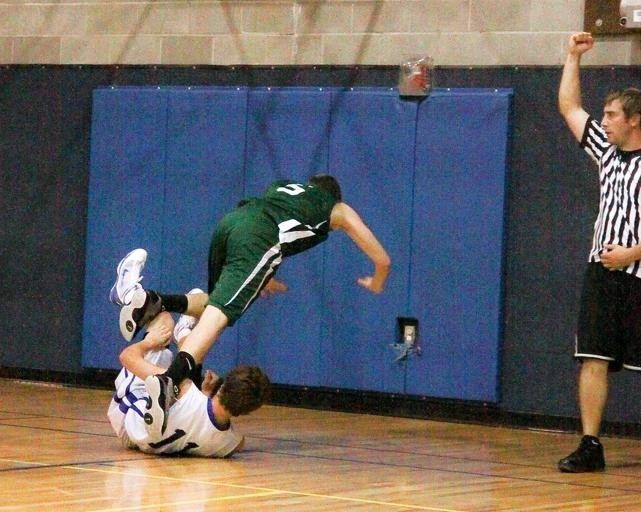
[
  {"xmin": 119, "ymin": 289, "xmax": 163, "ymax": 342},
  {"xmin": 143, "ymin": 373, "xmax": 181, "ymax": 440},
  {"xmin": 109, "ymin": 249, "xmax": 148, "ymax": 308},
  {"xmin": 558, "ymin": 436, "xmax": 606, "ymax": 473},
  {"xmin": 171, "ymin": 288, "xmax": 204, "ymax": 343}
]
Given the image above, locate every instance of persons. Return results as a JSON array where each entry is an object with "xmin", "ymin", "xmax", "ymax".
[
  {"xmin": 119, "ymin": 174, "xmax": 391, "ymax": 443},
  {"xmin": 558, "ymin": 31, "xmax": 641, "ymax": 473},
  {"xmin": 108, "ymin": 246, "xmax": 272, "ymax": 460}
]
